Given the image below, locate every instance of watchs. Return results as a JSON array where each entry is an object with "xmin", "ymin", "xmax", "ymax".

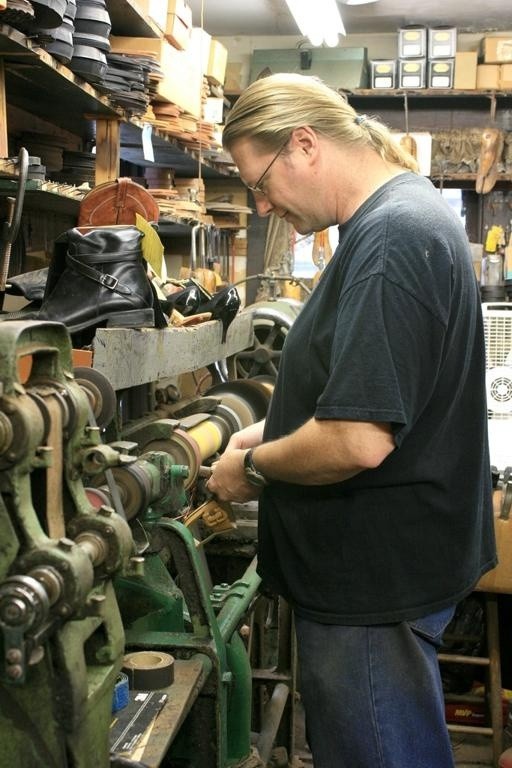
[{"xmin": 243, "ymin": 448, "xmax": 268, "ymax": 489}]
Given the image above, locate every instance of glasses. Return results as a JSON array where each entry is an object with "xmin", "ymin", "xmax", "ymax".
[{"xmin": 246, "ymin": 138, "xmax": 291, "ymax": 196}]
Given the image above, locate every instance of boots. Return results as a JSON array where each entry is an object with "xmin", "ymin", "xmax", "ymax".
[{"xmin": 41, "ymin": 229, "xmax": 167, "ymax": 332}]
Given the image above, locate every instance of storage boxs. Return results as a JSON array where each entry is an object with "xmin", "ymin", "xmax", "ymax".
[
  {"xmin": 248, "ymin": 24, "xmax": 512, "ymax": 90},
  {"xmin": 111, "ymin": 0, "xmax": 228, "ymax": 120}
]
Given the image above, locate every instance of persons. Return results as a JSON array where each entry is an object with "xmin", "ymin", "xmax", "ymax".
[{"xmin": 205, "ymin": 73, "xmax": 498, "ymax": 764}]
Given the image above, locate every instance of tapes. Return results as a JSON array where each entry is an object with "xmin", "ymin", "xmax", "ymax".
[{"xmin": 121, "ymin": 653, "xmax": 175, "ymax": 690}]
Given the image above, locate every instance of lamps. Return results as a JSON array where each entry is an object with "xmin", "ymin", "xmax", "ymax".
[{"xmin": 286, "ymin": 0, "xmax": 346, "ymax": 47}]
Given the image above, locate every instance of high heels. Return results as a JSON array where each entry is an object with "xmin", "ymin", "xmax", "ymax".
[
  {"xmin": 164, "ymin": 285, "xmax": 201, "ymax": 317},
  {"xmin": 199, "ymin": 287, "xmax": 240, "ymax": 345}
]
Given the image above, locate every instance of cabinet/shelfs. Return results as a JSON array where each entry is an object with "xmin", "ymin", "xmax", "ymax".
[{"xmin": 0, "ymin": 23, "xmax": 236, "ymax": 236}]
